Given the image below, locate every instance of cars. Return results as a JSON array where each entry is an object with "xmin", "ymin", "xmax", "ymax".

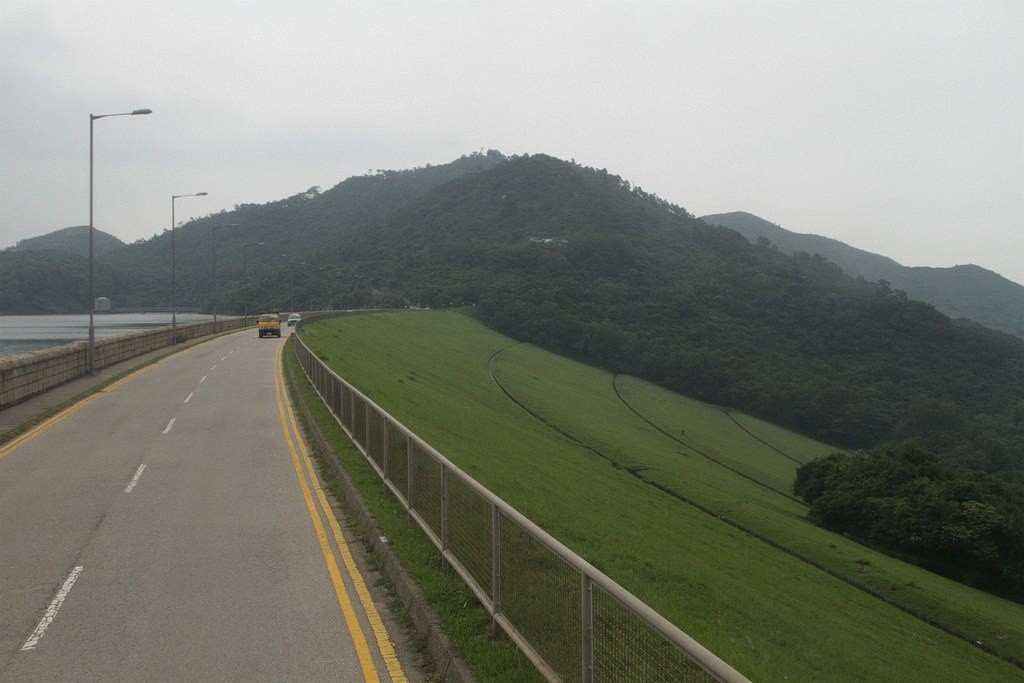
[
  {"xmin": 287, "ymin": 313, "xmax": 302, "ymax": 326},
  {"xmin": 256, "ymin": 313, "xmax": 281, "ymax": 338}
]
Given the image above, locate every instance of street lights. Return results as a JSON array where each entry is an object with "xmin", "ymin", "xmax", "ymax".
[
  {"xmin": 243, "ymin": 240, "xmax": 265, "ymax": 326},
  {"xmin": 171, "ymin": 191, "xmax": 210, "ymax": 344},
  {"xmin": 212, "ymin": 222, "xmax": 239, "ymax": 333},
  {"xmin": 88, "ymin": 108, "xmax": 154, "ymax": 376}
]
